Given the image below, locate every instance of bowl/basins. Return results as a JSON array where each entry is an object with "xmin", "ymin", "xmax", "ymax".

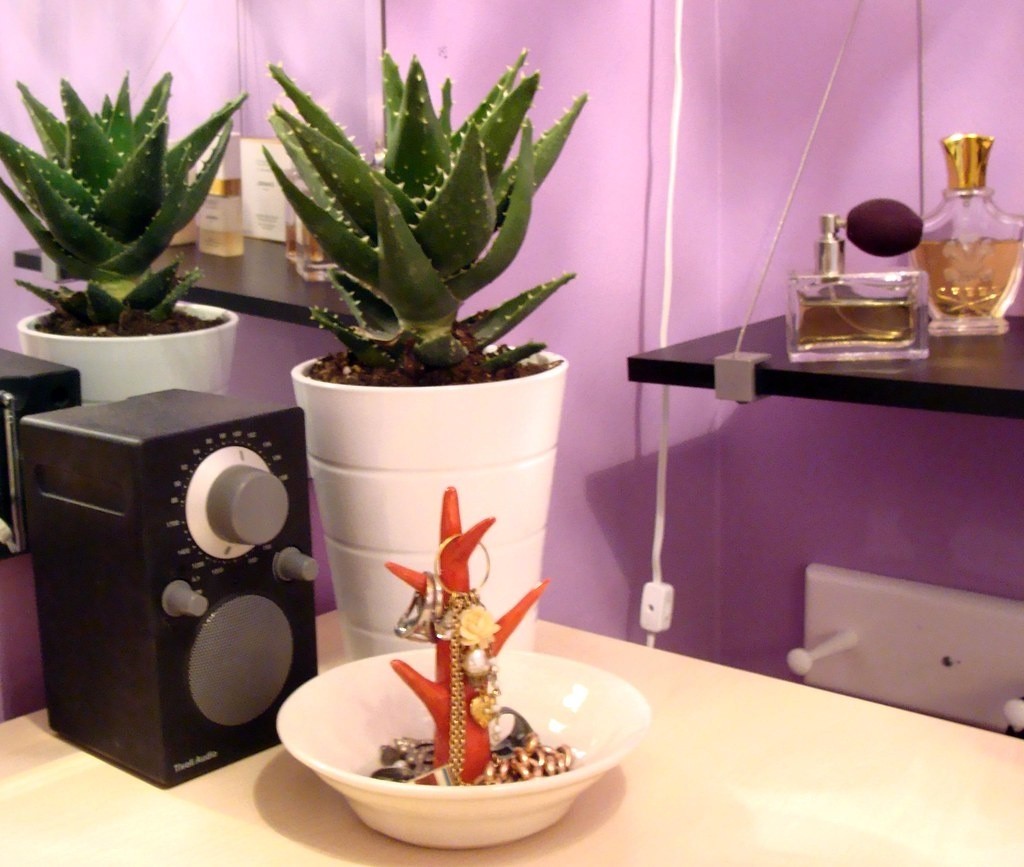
[{"xmin": 274, "ymin": 649, "xmax": 654, "ymax": 851}]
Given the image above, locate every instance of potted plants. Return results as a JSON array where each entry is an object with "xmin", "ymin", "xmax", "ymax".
[
  {"xmin": 259, "ymin": 48, "xmax": 592, "ymax": 663},
  {"xmin": 0, "ymin": 68, "xmax": 253, "ymax": 407}
]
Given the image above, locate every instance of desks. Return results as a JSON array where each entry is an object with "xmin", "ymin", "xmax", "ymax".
[
  {"xmin": 628, "ymin": 306, "xmax": 1023, "ymax": 419},
  {"xmin": 0, "ymin": 610, "xmax": 1024, "ymax": 867}
]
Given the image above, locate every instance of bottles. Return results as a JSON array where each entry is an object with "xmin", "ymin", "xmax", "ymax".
[
  {"xmin": 909, "ymin": 133, "xmax": 1024, "ymax": 337},
  {"xmin": 785, "ymin": 214, "xmax": 929, "ymax": 362},
  {"xmin": 167, "ymin": 132, "xmax": 387, "ymax": 282}
]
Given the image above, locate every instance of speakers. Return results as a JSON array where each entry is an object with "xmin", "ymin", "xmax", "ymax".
[{"xmin": 19, "ymin": 387, "xmax": 319, "ymax": 785}]
